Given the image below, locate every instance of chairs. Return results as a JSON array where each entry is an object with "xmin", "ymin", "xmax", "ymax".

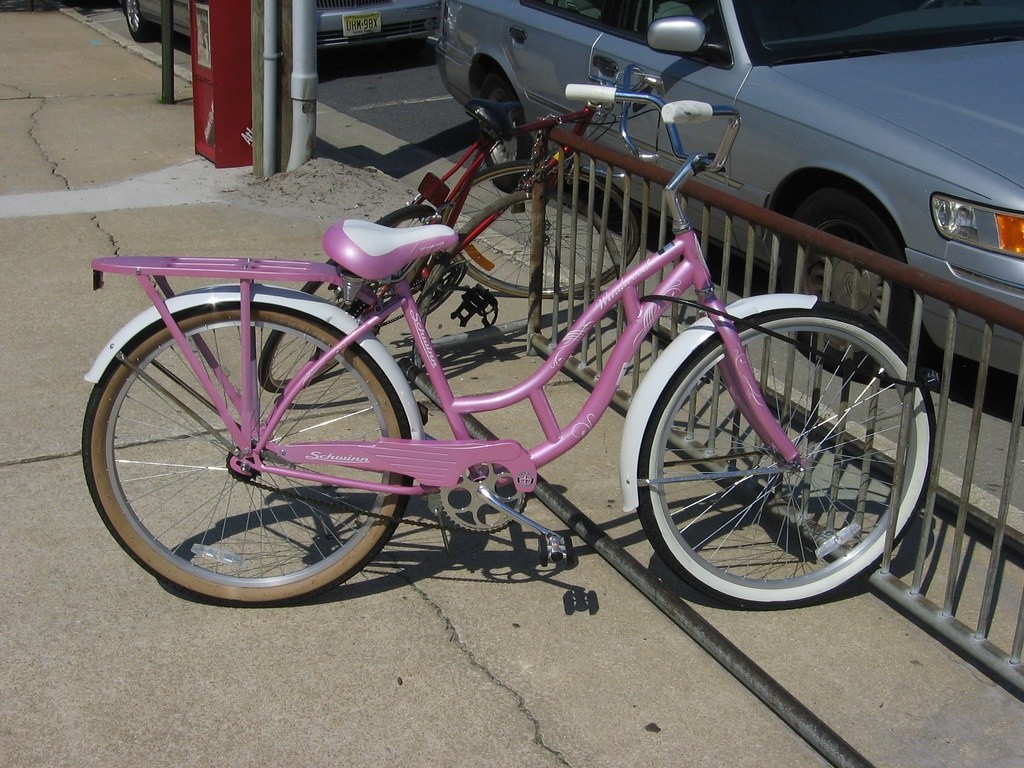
[{"xmin": 654, "ymin": 0, "xmax": 716, "ymax": 33}]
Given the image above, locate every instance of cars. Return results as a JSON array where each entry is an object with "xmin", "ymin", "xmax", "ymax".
[
  {"xmin": 123, "ymin": 0, "xmax": 441, "ymax": 53},
  {"xmin": 431, "ymin": 2, "xmax": 1024, "ymax": 380}
]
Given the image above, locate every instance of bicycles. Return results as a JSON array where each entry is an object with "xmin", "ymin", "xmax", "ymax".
[
  {"xmin": 75, "ymin": 78, "xmax": 935, "ymax": 610},
  {"xmin": 258, "ymin": 53, "xmax": 662, "ymax": 391}
]
